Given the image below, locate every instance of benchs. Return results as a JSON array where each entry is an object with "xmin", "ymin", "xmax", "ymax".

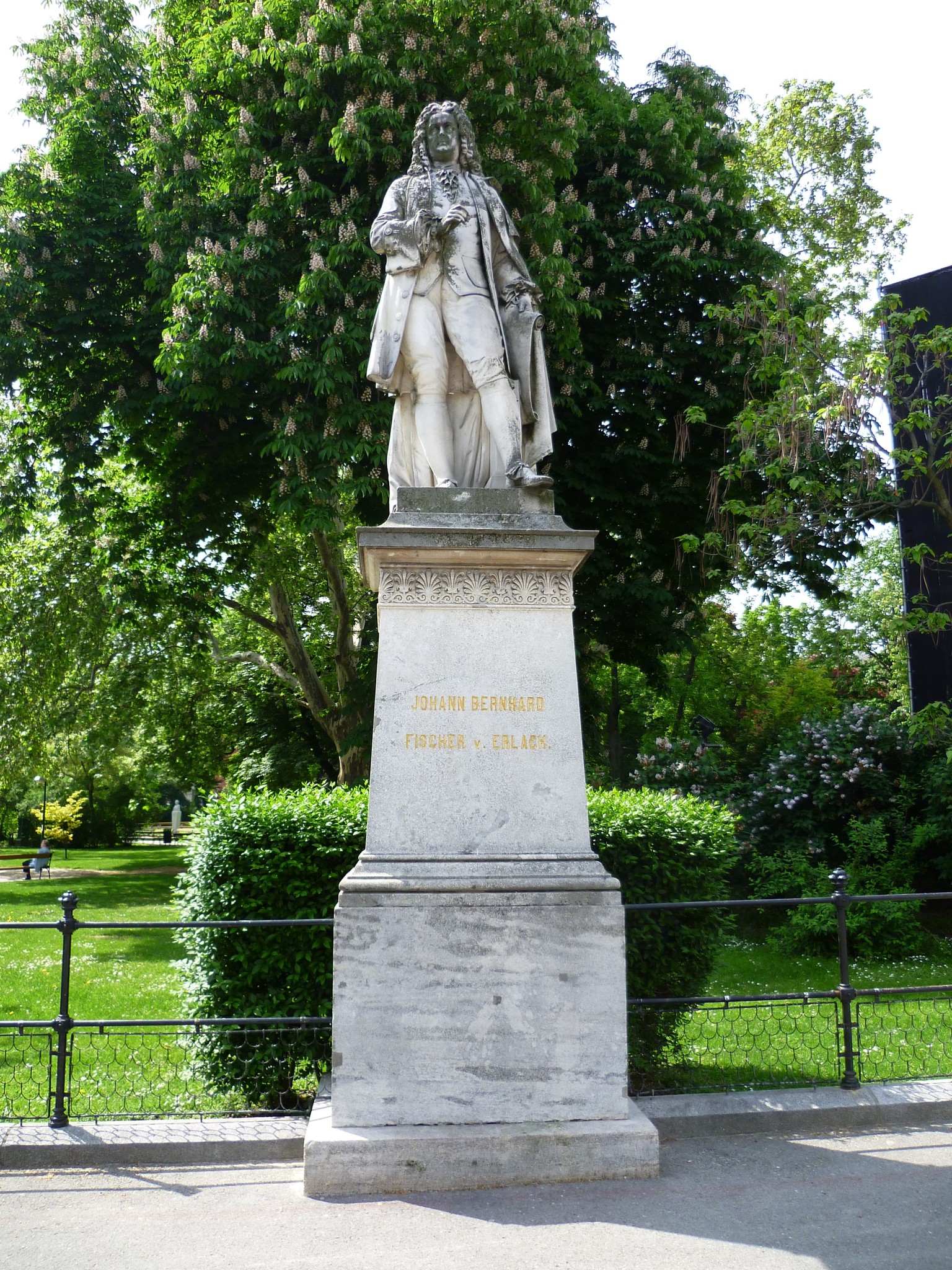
[
  {"xmin": 127, "ymin": 830, "xmax": 200, "ymax": 847},
  {"xmin": 0, "ymin": 852, "xmax": 54, "ymax": 880}
]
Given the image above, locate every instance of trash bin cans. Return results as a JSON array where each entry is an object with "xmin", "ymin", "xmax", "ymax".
[
  {"xmin": 163, "ymin": 828, "xmax": 171, "ymax": 843},
  {"xmin": 9, "ymin": 838, "xmax": 13, "ymax": 845}
]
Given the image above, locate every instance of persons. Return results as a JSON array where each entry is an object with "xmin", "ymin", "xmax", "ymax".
[
  {"xmin": 367, "ymin": 101, "xmax": 558, "ymax": 490},
  {"xmin": 173, "ymin": 800, "xmax": 180, "ymax": 810},
  {"xmin": 22, "ymin": 839, "xmax": 51, "ymax": 880}
]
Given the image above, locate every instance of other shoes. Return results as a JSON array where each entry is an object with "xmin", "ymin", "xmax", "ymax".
[{"xmin": 22, "ymin": 876, "xmax": 31, "ymax": 880}]
